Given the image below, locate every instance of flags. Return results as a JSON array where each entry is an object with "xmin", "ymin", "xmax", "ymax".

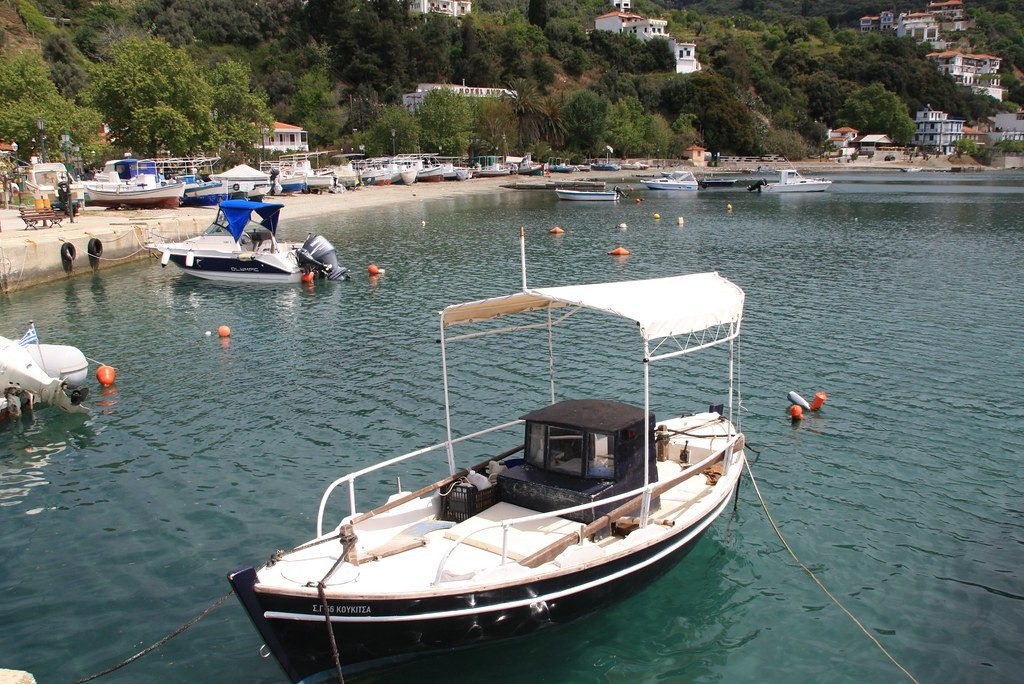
[{"xmin": 18, "ymin": 324, "xmax": 39, "ymax": 346}]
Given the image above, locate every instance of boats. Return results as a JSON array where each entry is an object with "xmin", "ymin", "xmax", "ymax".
[
  {"xmin": 555, "ymin": 184, "xmax": 629, "ymax": 201},
  {"xmin": 639, "ymin": 170, "xmax": 699, "ymax": 190},
  {"xmin": 0, "ymin": 333, "xmax": 92, "ymax": 421},
  {"xmin": 23, "ymin": 152, "xmax": 650, "ymax": 210},
  {"xmin": 144, "ymin": 199, "xmax": 350, "ymax": 283},
  {"xmin": 746, "ymin": 154, "xmax": 832, "ymax": 193},
  {"xmin": 226, "ymin": 223, "xmax": 748, "ymax": 684}
]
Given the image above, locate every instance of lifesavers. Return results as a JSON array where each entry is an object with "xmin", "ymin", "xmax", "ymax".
[
  {"xmin": 234, "ymin": 183, "xmax": 240, "ymax": 191},
  {"xmin": 88, "ymin": 238, "xmax": 104, "ymax": 258},
  {"xmin": 61, "ymin": 242, "xmax": 77, "ymax": 262}
]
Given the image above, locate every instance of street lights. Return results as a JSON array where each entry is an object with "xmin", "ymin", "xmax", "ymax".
[
  {"xmin": 258, "ymin": 124, "xmax": 268, "ymax": 160},
  {"xmin": 494, "ymin": 145, "xmax": 499, "ymax": 156},
  {"xmin": 10, "ymin": 141, "xmax": 21, "ymax": 205},
  {"xmin": 59, "ymin": 128, "xmax": 71, "ymax": 169},
  {"xmin": 390, "ymin": 128, "xmax": 396, "ymax": 158},
  {"xmin": 358, "ymin": 141, "xmax": 365, "ymax": 160},
  {"xmin": 33, "ymin": 117, "xmax": 45, "ymax": 163},
  {"xmin": 439, "ymin": 144, "xmax": 442, "ymax": 154},
  {"xmin": 74, "ymin": 143, "xmax": 80, "ymax": 176}
]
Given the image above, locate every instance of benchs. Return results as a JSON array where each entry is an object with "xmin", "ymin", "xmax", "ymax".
[{"xmin": 18, "ymin": 207, "xmax": 64, "ymax": 230}]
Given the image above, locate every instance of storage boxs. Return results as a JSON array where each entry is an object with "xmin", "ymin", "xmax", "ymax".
[{"xmin": 439, "ymin": 467, "xmax": 502, "ymax": 523}]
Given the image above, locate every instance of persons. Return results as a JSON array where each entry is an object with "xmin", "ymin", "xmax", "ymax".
[{"xmin": 361, "ymin": 166, "xmax": 366, "ymax": 173}]
